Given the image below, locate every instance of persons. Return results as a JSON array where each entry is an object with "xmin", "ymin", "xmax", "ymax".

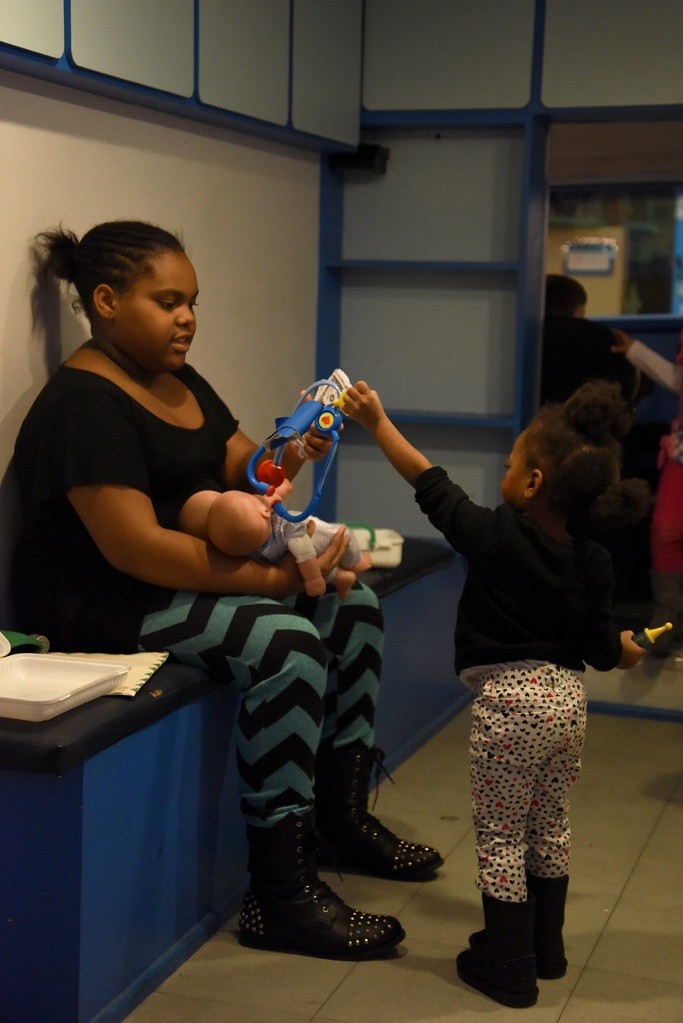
[
  {"xmin": 545, "ymin": 274, "xmax": 588, "ymax": 316},
  {"xmin": 0, "ymin": 220, "xmax": 446, "ymax": 962},
  {"xmin": 207, "ymin": 476, "xmax": 372, "ymax": 602},
  {"xmin": 177, "ymin": 490, "xmax": 221, "ymax": 540},
  {"xmin": 342, "ymin": 380, "xmax": 650, "ymax": 1008},
  {"xmin": 612, "ymin": 328, "xmax": 683, "ymax": 655}
]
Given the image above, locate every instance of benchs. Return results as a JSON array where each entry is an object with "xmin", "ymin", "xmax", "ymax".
[{"xmin": 0, "ymin": 540, "xmax": 478, "ymax": 1023}]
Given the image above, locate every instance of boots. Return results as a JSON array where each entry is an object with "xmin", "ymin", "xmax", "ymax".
[
  {"xmin": 456, "ymin": 890, "xmax": 540, "ymax": 1009},
  {"xmin": 646, "ymin": 568, "xmax": 683, "ymax": 651},
  {"xmin": 311, "ymin": 742, "xmax": 445, "ymax": 880},
  {"xmin": 468, "ymin": 869, "xmax": 568, "ymax": 981},
  {"xmin": 237, "ymin": 814, "xmax": 407, "ymax": 954}
]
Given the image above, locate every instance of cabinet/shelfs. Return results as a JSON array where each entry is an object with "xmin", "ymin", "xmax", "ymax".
[{"xmin": 0, "ymin": 0, "xmax": 683, "ymax": 536}]
